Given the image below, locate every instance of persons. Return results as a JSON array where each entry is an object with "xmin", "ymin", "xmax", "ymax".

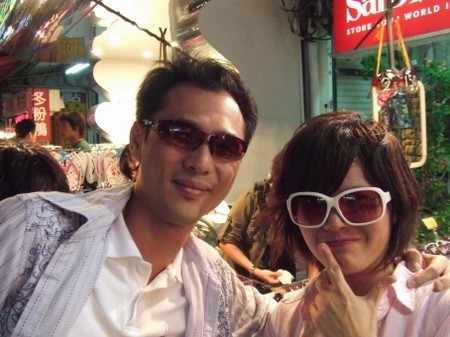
[{"xmin": 1, "ymin": 52, "xmax": 450, "ymax": 337}]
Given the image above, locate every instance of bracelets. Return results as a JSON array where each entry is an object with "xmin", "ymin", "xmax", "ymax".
[{"xmin": 250, "ymin": 266, "xmax": 257, "ymax": 276}]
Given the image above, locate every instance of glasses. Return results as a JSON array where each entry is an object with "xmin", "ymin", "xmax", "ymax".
[
  {"xmin": 141, "ymin": 118, "xmax": 247, "ymax": 163},
  {"xmin": 287, "ymin": 187, "xmax": 392, "ymax": 228}
]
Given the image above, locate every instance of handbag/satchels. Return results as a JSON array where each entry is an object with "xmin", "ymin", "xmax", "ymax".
[{"xmin": 372, "ymin": 11, "xmax": 428, "ymax": 169}]
